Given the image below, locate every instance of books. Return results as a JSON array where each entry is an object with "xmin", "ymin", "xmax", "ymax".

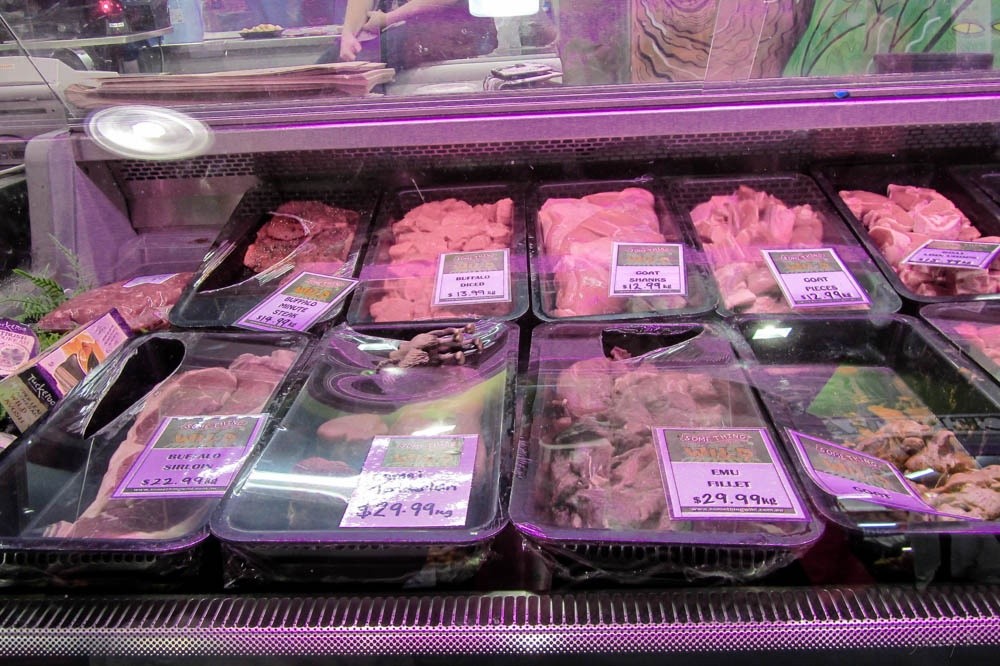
[{"xmin": 64, "ymin": 60, "xmax": 396, "ymax": 107}]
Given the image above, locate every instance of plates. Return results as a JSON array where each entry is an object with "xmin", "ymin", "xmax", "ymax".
[{"xmin": 240, "ymin": 28, "xmax": 283, "ymax": 38}]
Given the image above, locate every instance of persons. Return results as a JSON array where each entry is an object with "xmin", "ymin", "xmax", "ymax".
[{"xmin": 339, "ymin": 1, "xmax": 495, "ymax": 70}]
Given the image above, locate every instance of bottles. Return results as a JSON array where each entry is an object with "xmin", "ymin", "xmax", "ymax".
[{"xmin": 120, "ymin": 47, "xmax": 141, "ymax": 72}]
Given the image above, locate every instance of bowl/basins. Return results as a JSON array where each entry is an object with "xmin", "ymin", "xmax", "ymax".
[{"xmin": 873, "ymin": 51, "xmax": 993, "ymax": 73}]
